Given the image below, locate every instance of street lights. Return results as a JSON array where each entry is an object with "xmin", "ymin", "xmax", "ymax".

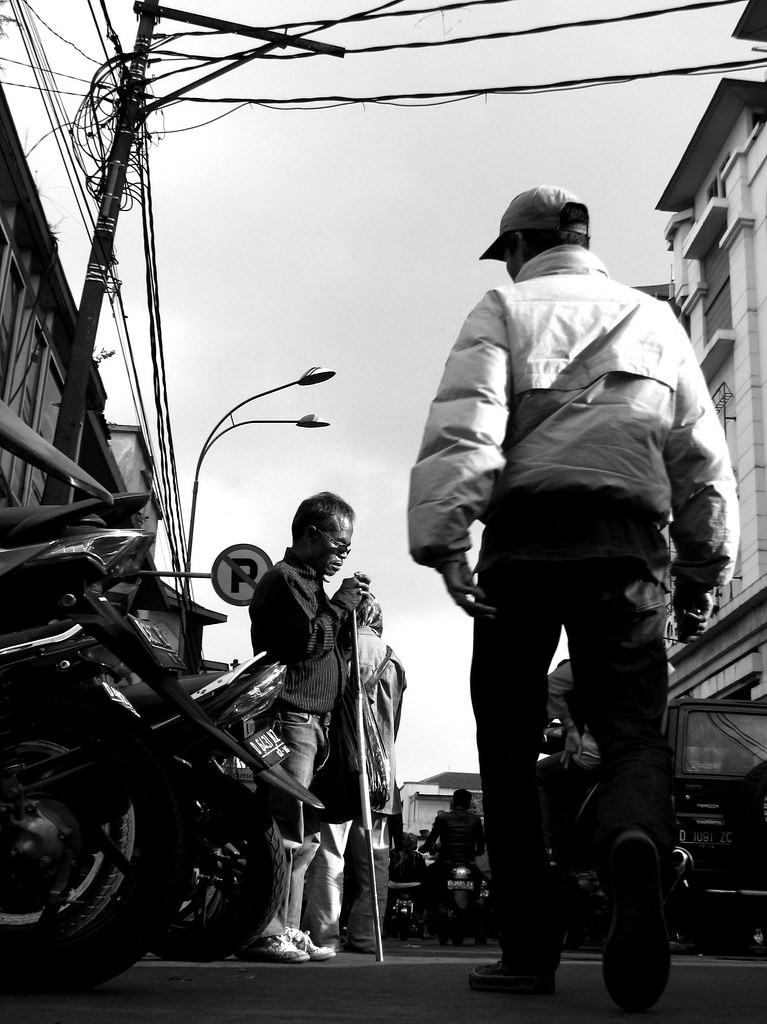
[{"xmin": 178, "ymin": 363, "xmax": 337, "ymax": 659}]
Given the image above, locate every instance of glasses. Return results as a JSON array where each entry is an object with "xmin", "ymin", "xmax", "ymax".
[{"xmin": 316, "ymin": 529, "xmax": 352, "ymax": 555}]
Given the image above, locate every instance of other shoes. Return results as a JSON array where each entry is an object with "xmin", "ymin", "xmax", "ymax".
[
  {"xmin": 600, "ymin": 830, "xmax": 672, "ymax": 1014},
  {"xmin": 469, "ymin": 959, "xmax": 557, "ymax": 996}
]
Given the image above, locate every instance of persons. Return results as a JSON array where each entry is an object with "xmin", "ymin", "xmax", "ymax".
[
  {"xmin": 418, "ymin": 789, "xmax": 485, "ymax": 912},
  {"xmin": 535, "ymin": 656, "xmax": 603, "ymax": 868},
  {"xmin": 298, "ymin": 589, "xmax": 407, "ymax": 955},
  {"xmin": 382, "ymin": 832, "xmax": 427, "ymax": 942},
  {"xmin": 406, "ymin": 186, "xmax": 742, "ymax": 1009},
  {"xmin": 249, "ymin": 490, "xmax": 370, "ymax": 964}
]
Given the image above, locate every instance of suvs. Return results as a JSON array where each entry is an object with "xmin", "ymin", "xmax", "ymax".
[{"xmin": 658, "ymin": 695, "xmax": 767, "ymax": 957}]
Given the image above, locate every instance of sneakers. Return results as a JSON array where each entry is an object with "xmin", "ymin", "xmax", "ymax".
[
  {"xmin": 234, "ymin": 934, "xmax": 311, "ymax": 963},
  {"xmin": 283, "ymin": 927, "xmax": 336, "ymax": 960}
]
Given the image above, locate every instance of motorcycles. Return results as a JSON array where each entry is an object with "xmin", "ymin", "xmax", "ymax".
[
  {"xmin": 100, "ymin": 650, "xmax": 328, "ymax": 965},
  {"xmin": 420, "ymin": 828, "xmax": 485, "ymax": 945},
  {"xmin": 0, "ymin": 491, "xmax": 269, "ymax": 995},
  {"xmin": 390, "ymin": 886, "xmax": 418, "ymax": 940},
  {"xmin": 540, "ymin": 723, "xmax": 697, "ymax": 950}
]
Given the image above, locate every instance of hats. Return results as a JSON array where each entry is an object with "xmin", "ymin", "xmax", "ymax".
[{"xmin": 478, "ymin": 185, "xmax": 592, "ymax": 263}]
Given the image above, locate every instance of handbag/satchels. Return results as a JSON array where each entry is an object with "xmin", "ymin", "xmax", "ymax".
[{"xmin": 318, "ymin": 676, "xmax": 391, "ymax": 824}]
